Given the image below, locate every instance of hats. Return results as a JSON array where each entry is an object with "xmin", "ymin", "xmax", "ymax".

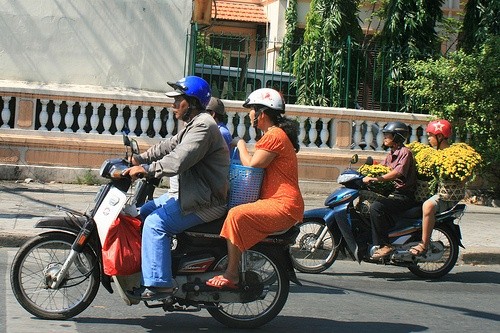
[
  {"xmin": 206, "ymin": 97, "xmax": 225, "ymax": 116},
  {"xmin": 164, "ymin": 88, "xmax": 184, "ymax": 97}
]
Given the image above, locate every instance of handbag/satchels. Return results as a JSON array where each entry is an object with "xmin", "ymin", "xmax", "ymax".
[{"xmin": 102, "ymin": 213, "xmax": 142, "ymax": 275}]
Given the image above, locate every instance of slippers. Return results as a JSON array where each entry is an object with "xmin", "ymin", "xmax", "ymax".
[
  {"xmin": 126, "ymin": 288, "xmax": 173, "ymax": 299},
  {"xmin": 206, "ymin": 276, "xmax": 240, "ymax": 290}
]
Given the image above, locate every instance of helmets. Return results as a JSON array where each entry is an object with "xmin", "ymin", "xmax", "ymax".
[
  {"xmin": 167, "ymin": 76, "xmax": 211, "ymax": 110},
  {"xmin": 242, "ymin": 87, "xmax": 285, "ymax": 114},
  {"xmin": 381, "ymin": 121, "xmax": 409, "ymax": 138},
  {"xmin": 426, "ymin": 118, "xmax": 452, "ymax": 139}
]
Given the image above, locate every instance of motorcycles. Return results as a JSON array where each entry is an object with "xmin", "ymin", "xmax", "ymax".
[
  {"xmin": 285, "ymin": 152, "xmax": 466, "ymax": 279},
  {"xmin": 10, "ymin": 130, "xmax": 304, "ymax": 329}
]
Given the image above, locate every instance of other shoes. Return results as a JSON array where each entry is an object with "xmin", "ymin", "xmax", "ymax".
[{"xmin": 371, "ymin": 244, "xmax": 396, "ymax": 258}]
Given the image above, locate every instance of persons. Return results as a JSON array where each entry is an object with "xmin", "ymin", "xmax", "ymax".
[
  {"xmin": 206, "ymin": 95, "xmax": 234, "ymax": 150},
  {"xmin": 123, "ymin": 76, "xmax": 231, "ymax": 299},
  {"xmin": 360, "ymin": 121, "xmax": 418, "ymax": 259},
  {"xmin": 204, "ymin": 89, "xmax": 304, "ymax": 289},
  {"xmin": 407, "ymin": 119, "xmax": 466, "ymax": 256}
]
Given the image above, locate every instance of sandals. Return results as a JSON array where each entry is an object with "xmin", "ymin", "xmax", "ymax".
[{"xmin": 409, "ymin": 241, "xmax": 431, "ymax": 256}]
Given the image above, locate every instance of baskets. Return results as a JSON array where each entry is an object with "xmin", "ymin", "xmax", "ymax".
[
  {"xmin": 358, "ymin": 190, "xmax": 389, "ymax": 215},
  {"xmin": 438, "ymin": 182, "xmax": 466, "ymax": 201},
  {"xmin": 226, "ymin": 147, "xmax": 265, "ymax": 209},
  {"xmin": 413, "ymin": 175, "xmax": 437, "ymax": 204}
]
